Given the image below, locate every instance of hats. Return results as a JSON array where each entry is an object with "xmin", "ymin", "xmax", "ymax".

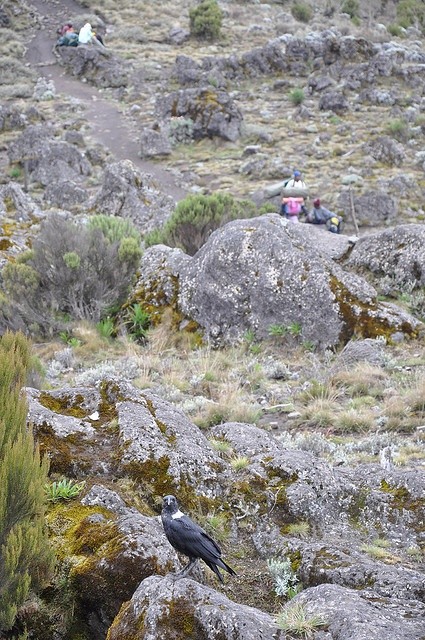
[
  {"xmin": 295, "ymin": 171, "xmax": 300, "ymax": 176},
  {"xmin": 313, "ymin": 198, "xmax": 320, "ymax": 205}
]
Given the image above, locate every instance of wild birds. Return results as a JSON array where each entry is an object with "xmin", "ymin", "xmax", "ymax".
[{"xmin": 160, "ymin": 494, "xmax": 237, "ymax": 586}]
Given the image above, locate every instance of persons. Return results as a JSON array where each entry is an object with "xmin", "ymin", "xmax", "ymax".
[
  {"xmin": 57, "ymin": 31, "xmax": 79, "ymax": 47},
  {"xmin": 280, "ymin": 170, "xmax": 308, "ymax": 217},
  {"xmin": 60, "ymin": 23, "xmax": 72, "ymax": 35},
  {"xmin": 303, "ymin": 197, "xmax": 339, "ymax": 225},
  {"xmin": 78, "ymin": 22, "xmax": 103, "ymax": 44}
]
